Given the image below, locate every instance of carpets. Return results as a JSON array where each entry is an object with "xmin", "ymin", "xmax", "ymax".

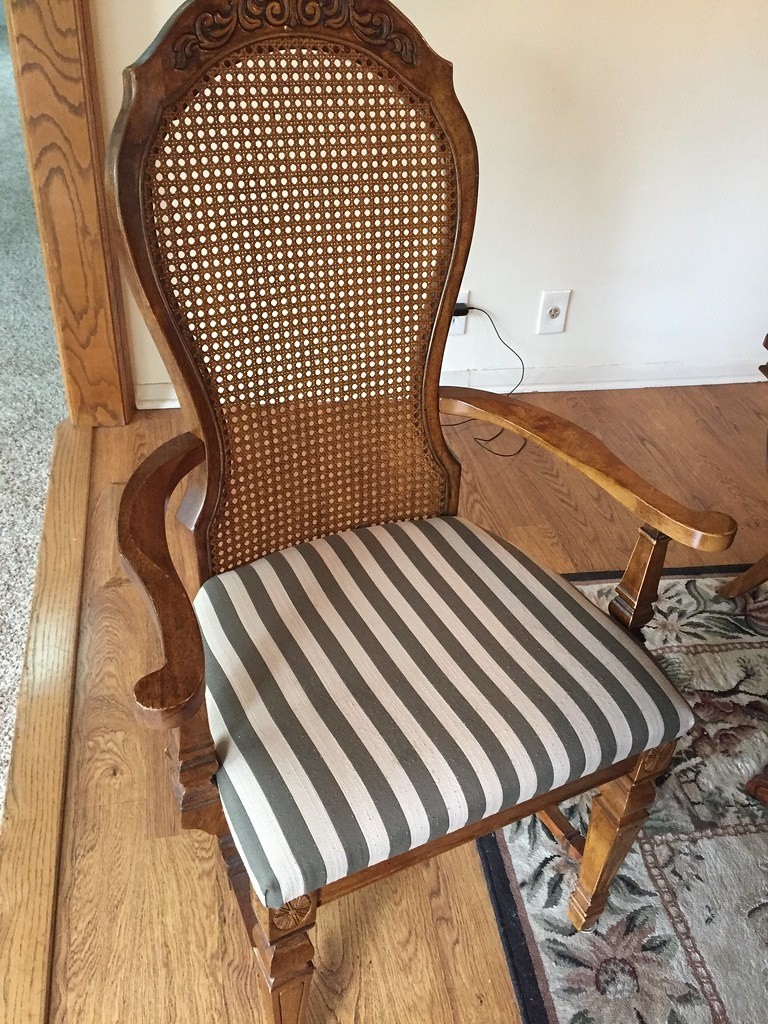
[{"xmin": 474, "ymin": 565, "xmax": 767, "ymax": 1024}]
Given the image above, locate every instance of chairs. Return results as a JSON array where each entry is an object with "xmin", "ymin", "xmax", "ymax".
[{"xmin": 116, "ymin": 0, "xmax": 736, "ymax": 1024}]
